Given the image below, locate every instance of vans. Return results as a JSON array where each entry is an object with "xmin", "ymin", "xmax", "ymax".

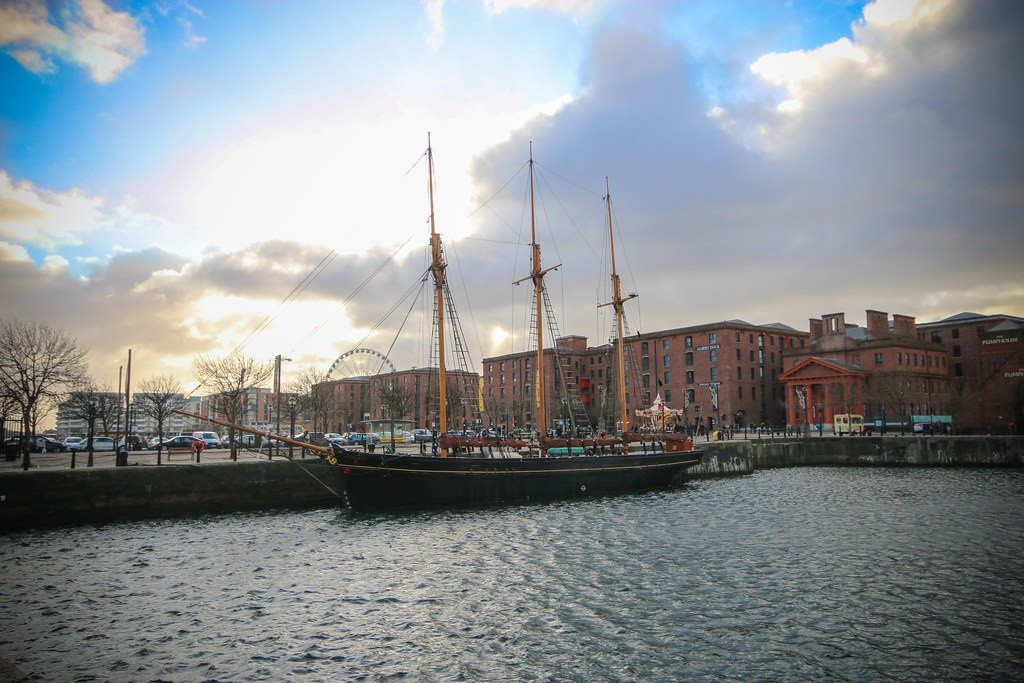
[{"xmin": 193, "ymin": 432, "xmax": 221, "ymax": 449}]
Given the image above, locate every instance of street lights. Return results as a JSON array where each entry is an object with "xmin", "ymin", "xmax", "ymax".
[
  {"xmin": 128, "ymin": 403, "xmax": 134, "ymax": 451},
  {"xmin": 802, "ymin": 387, "xmax": 808, "ymax": 424},
  {"xmin": 682, "ymin": 389, "xmax": 686, "ymax": 417},
  {"xmin": 461, "ymin": 399, "xmax": 467, "ymax": 452},
  {"xmin": 288, "ymin": 397, "xmax": 297, "ymax": 459},
  {"xmin": 598, "ymin": 385, "xmax": 603, "ymax": 418},
  {"xmin": 561, "ymin": 398, "xmax": 568, "ymax": 438},
  {"xmin": 661, "ymin": 400, "xmax": 665, "ymax": 431},
  {"xmin": 276, "ymin": 355, "xmax": 292, "ymax": 455},
  {"xmin": 87, "ymin": 396, "xmax": 100, "ymax": 467},
  {"xmin": 267, "ymin": 404, "xmax": 273, "ymax": 424},
  {"xmin": 505, "ymin": 409, "xmax": 510, "ymax": 439},
  {"xmin": 381, "ymin": 405, "xmax": 386, "ymax": 436}
]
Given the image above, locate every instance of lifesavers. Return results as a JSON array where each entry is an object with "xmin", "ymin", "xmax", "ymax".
[{"xmin": 192, "ymin": 441, "xmax": 205, "ymax": 452}]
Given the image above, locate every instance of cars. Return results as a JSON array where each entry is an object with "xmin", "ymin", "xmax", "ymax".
[
  {"xmin": 221, "ymin": 435, "xmax": 275, "ymax": 448},
  {"xmin": 308, "ymin": 429, "xmax": 433, "ymax": 446},
  {"xmin": 147, "ymin": 436, "xmax": 205, "ymax": 450},
  {"xmin": 4, "ymin": 435, "xmax": 143, "ymax": 453}
]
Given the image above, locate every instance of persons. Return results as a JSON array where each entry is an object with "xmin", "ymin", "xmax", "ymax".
[
  {"xmin": 482, "ymin": 435, "xmax": 486, "ymax": 442},
  {"xmin": 599, "ymin": 427, "xmax": 606, "ymax": 454},
  {"xmin": 868, "ymin": 428, "xmax": 871, "ymax": 436},
  {"xmin": 750, "ymin": 421, "xmax": 753, "ymax": 432},
  {"xmin": 863, "ymin": 427, "xmax": 866, "ymax": 436},
  {"xmin": 713, "ymin": 429, "xmax": 721, "ymax": 441},
  {"xmin": 191, "ymin": 437, "xmax": 204, "ymax": 453},
  {"xmin": 635, "ymin": 425, "xmax": 638, "ymax": 430},
  {"xmin": 761, "ymin": 423, "xmax": 765, "ymax": 434},
  {"xmin": 38, "ymin": 440, "xmax": 45, "ymax": 452},
  {"xmin": 304, "ymin": 431, "xmax": 311, "ymax": 453},
  {"xmin": 754, "ymin": 424, "xmax": 757, "ymax": 433},
  {"xmin": 700, "ymin": 422, "xmax": 704, "ymax": 436}
]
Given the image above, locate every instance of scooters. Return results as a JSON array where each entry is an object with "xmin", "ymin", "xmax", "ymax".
[{"xmin": 834, "ymin": 414, "xmax": 865, "ymax": 436}]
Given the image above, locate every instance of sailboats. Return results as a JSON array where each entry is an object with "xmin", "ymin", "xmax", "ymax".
[{"xmin": 170, "ymin": 133, "xmax": 706, "ymax": 514}]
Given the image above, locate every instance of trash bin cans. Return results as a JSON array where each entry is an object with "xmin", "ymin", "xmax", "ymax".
[
  {"xmin": 117, "ymin": 452, "xmax": 128, "ymax": 466},
  {"xmin": 4, "ymin": 443, "xmax": 18, "ymax": 461}
]
